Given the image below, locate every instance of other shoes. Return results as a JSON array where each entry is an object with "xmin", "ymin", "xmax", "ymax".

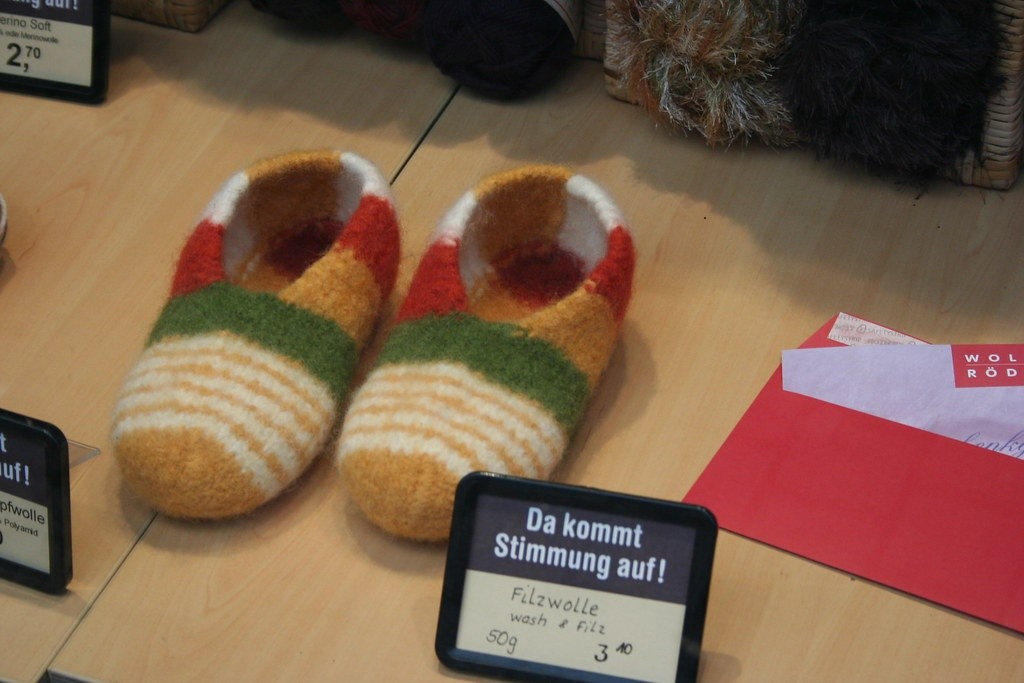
[
  {"xmin": 110, "ymin": 151, "xmax": 402, "ymax": 522},
  {"xmin": 338, "ymin": 166, "xmax": 638, "ymax": 543}
]
